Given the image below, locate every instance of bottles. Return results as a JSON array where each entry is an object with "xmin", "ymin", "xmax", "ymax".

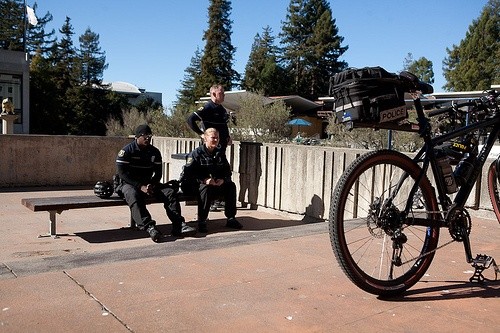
[
  {"xmin": 452, "ymin": 152, "xmax": 478, "ymax": 186},
  {"xmin": 433, "ymin": 145, "xmax": 458, "ymax": 194}
]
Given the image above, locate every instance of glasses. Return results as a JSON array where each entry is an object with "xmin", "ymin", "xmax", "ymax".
[{"xmin": 143, "ymin": 135, "xmax": 152, "ymax": 139}]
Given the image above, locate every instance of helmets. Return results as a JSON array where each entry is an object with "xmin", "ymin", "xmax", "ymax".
[{"xmin": 94, "ymin": 181, "xmax": 114, "ymax": 199}]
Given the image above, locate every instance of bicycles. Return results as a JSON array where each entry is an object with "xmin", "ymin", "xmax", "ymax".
[{"xmin": 327, "ymin": 71, "xmax": 500, "ymax": 298}]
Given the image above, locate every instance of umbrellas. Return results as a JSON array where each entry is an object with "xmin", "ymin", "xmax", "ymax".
[{"xmin": 288, "ymin": 117, "xmax": 312, "ymax": 132}]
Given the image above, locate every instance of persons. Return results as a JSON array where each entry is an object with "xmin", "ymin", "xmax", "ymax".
[
  {"xmin": 113, "ymin": 125, "xmax": 196, "ymax": 241},
  {"xmin": 187, "ymin": 84, "xmax": 231, "ymax": 212},
  {"xmin": 179, "ymin": 128, "xmax": 244, "ymax": 234}
]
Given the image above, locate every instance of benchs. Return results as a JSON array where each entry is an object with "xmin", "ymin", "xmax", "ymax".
[{"xmin": 22, "ymin": 193, "xmax": 196, "ymax": 239}]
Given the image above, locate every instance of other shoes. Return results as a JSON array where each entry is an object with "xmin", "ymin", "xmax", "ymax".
[
  {"xmin": 227, "ymin": 218, "xmax": 244, "ymax": 230},
  {"xmin": 198, "ymin": 222, "xmax": 208, "ymax": 233},
  {"xmin": 175, "ymin": 222, "xmax": 196, "ymax": 234},
  {"xmin": 148, "ymin": 225, "xmax": 161, "ymax": 242}
]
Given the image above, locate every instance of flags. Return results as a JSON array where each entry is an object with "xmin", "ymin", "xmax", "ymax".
[{"xmin": 26, "ymin": 6, "xmax": 38, "ymax": 26}]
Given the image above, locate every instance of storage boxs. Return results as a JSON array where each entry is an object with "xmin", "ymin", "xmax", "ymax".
[{"xmin": 330, "ymin": 67, "xmax": 409, "ymax": 123}]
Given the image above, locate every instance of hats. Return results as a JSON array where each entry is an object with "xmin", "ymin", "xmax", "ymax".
[{"xmin": 136, "ymin": 124, "xmax": 152, "ymax": 139}]
